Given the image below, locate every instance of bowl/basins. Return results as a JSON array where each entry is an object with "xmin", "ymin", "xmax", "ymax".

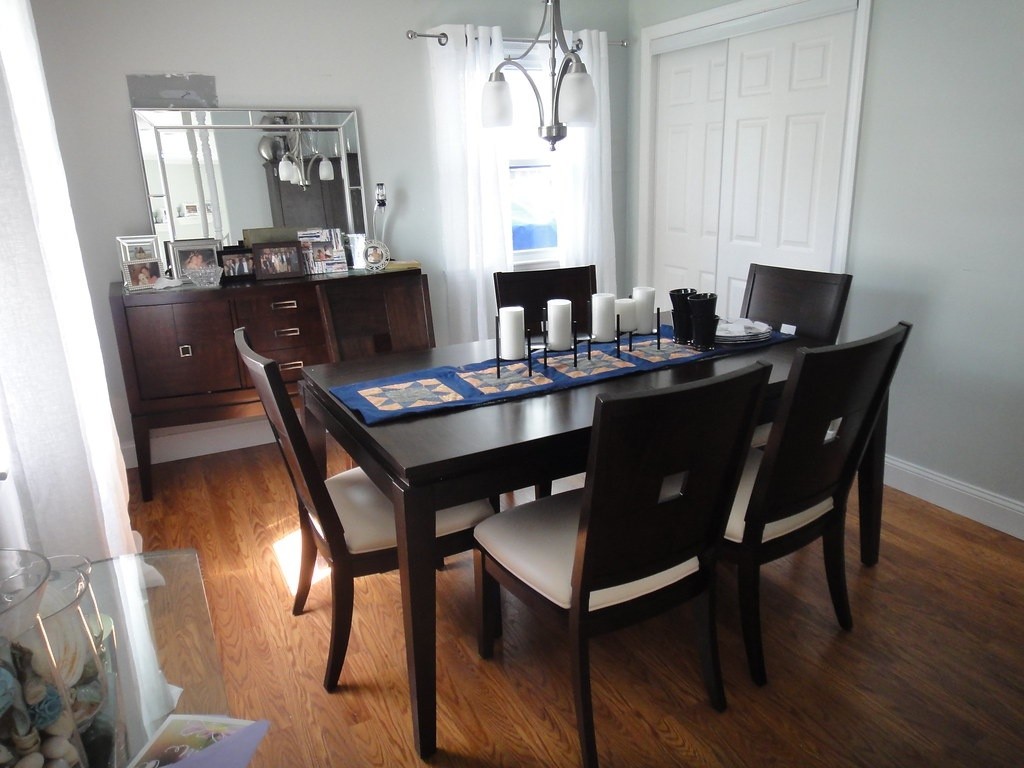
[{"xmin": 182, "ymin": 264, "xmax": 223, "ymax": 288}]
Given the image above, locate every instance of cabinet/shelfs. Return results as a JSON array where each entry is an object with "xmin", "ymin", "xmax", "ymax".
[{"xmin": 111, "ymin": 272, "xmax": 435, "ymax": 417}]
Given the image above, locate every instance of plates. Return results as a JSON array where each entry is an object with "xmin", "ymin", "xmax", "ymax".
[{"xmin": 715, "ymin": 319, "xmax": 773, "ymax": 344}]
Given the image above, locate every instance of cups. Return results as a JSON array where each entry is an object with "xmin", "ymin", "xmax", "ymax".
[{"xmin": 669, "ymin": 288, "xmax": 720, "ymax": 352}]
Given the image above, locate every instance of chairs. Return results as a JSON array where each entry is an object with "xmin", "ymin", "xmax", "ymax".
[
  {"xmin": 233, "ymin": 325, "xmax": 504, "ymax": 693},
  {"xmin": 741, "ymin": 263, "xmax": 853, "ymax": 345},
  {"xmin": 492, "ymin": 262, "xmax": 597, "ymax": 498},
  {"xmin": 473, "ymin": 361, "xmax": 773, "ymax": 768},
  {"xmin": 720, "ymin": 319, "xmax": 913, "ymax": 688}
]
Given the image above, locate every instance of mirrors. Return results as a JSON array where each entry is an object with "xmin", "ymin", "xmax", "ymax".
[{"xmin": 133, "ymin": 106, "xmax": 369, "ymax": 275}]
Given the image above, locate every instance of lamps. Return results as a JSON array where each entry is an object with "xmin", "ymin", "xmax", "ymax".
[
  {"xmin": 480, "ymin": 0, "xmax": 599, "ymax": 151},
  {"xmin": 278, "ymin": 114, "xmax": 334, "ymax": 190}
]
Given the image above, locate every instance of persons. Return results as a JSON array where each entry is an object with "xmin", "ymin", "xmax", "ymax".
[
  {"xmin": 261, "ymin": 249, "xmax": 297, "ymax": 274},
  {"xmin": 324, "ymin": 246, "xmax": 332, "ymax": 259},
  {"xmin": 135, "ymin": 247, "xmax": 145, "ymax": 258},
  {"xmin": 140, "ymin": 267, "xmax": 157, "ymax": 284},
  {"xmin": 316, "ymin": 250, "xmax": 324, "ymax": 261},
  {"xmin": 370, "ymin": 248, "xmax": 381, "ymax": 261},
  {"xmin": 224, "ymin": 256, "xmax": 255, "ymax": 276},
  {"xmin": 184, "ymin": 250, "xmax": 203, "ymax": 268}
]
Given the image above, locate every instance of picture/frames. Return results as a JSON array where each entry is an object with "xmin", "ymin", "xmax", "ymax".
[
  {"xmin": 167, "ymin": 240, "xmax": 222, "ymax": 284},
  {"xmin": 123, "ymin": 258, "xmax": 166, "ymax": 291},
  {"xmin": 250, "ymin": 241, "xmax": 306, "ymax": 279},
  {"xmin": 216, "ymin": 248, "xmax": 256, "ymax": 279},
  {"xmin": 173, "ymin": 245, "xmax": 218, "ymax": 279},
  {"xmin": 116, "ymin": 235, "xmax": 161, "ymax": 288}
]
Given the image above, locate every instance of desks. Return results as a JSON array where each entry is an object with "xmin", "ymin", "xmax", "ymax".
[{"xmin": 295, "ymin": 310, "xmax": 888, "ymax": 760}]
